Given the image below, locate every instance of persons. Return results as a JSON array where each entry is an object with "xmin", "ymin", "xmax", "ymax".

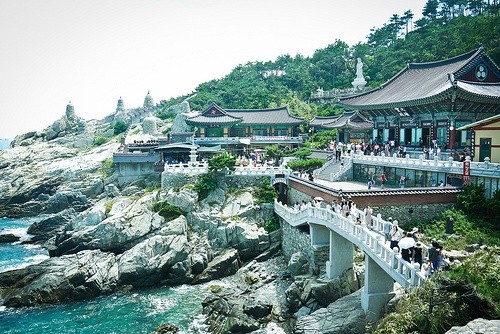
[
  {"xmin": 428, "ymin": 241, "xmax": 444, "ymax": 271},
  {"xmin": 372, "ymin": 172, "xmax": 377, "ymax": 185},
  {"xmin": 378, "ymin": 135, "xmax": 382, "ymax": 145},
  {"xmin": 356, "ymin": 58, "xmax": 363, "ymax": 78},
  {"xmin": 400, "ymin": 176, "xmax": 406, "ymax": 188},
  {"xmin": 367, "ymin": 178, "xmax": 372, "ymax": 190},
  {"xmin": 326, "ymin": 197, "xmax": 362, "ymax": 225},
  {"xmin": 384, "ymin": 219, "xmax": 417, "ymax": 263},
  {"xmin": 381, "ymin": 173, "xmax": 387, "ymax": 188},
  {"xmin": 439, "ymin": 180, "xmax": 444, "ymax": 187},
  {"xmin": 454, "ymin": 144, "xmax": 471, "ymax": 161},
  {"xmin": 413, "ymin": 243, "xmax": 422, "ymax": 269},
  {"xmin": 294, "ymin": 200, "xmax": 322, "ymax": 210},
  {"xmin": 364, "ymin": 205, "xmax": 372, "ymax": 230},
  {"xmin": 444, "ymin": 256, "xmax": 456, "ymax": 271},
  {"xmin": 419, "ymin": 137, "xmax": 447, "ymax": 159},
  {"xmin": 330, "ymin": 137, "xmax": 406, "ymax": 158}
]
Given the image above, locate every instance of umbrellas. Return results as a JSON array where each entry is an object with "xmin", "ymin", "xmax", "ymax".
[
  {"xmin": 313, "ymin": 197, "xmax": 324, "ymax": 200},
  {"xmin": 397, "ymin": 237, "xmax": 415, "ymax": 250}
]
